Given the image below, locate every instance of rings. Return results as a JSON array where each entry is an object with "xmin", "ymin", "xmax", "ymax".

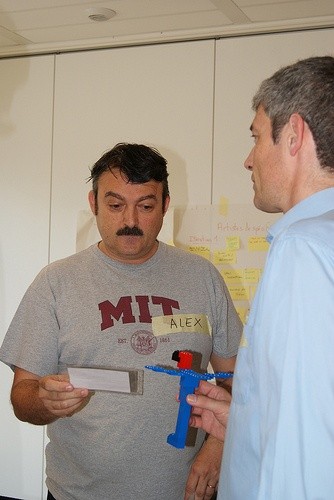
[{"xmin": 207, "ymin": 485, "xmax": 215, "ymax": 488}]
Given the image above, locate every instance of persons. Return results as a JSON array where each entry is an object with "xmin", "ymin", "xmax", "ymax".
[
  {"xmin": 0, "ymin": 143, "xmax": 243, "ymax": 500},
  {"xmin": 172, "ymin": 55, "xmax": 334, "ymax": 500}
]
